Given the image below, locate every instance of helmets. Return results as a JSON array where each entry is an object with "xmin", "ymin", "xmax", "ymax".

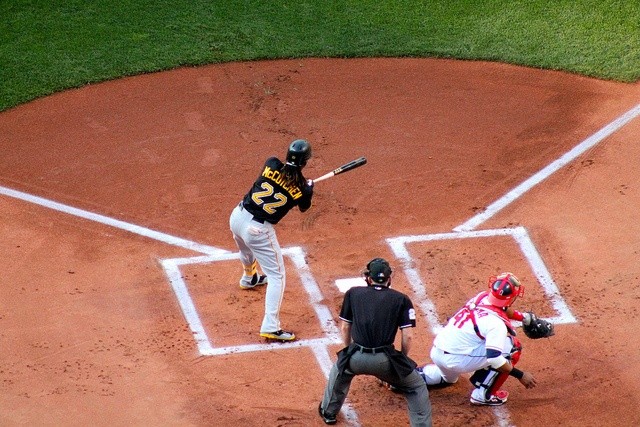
[
  {"xmin": 287, "ymin": 140, "xmax": 312, "ymax": 168},
  {"xmin": 365, "ymin": 258, "xmax": 393, "ymax": 293},
  {"xmin": 488, "ymin": 272, "xmax": 525, "ymax": 307}
]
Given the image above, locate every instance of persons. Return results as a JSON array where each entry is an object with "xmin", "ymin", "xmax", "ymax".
[
  {"xmin": 379, "ymin": 273, "xmax": 536, "ymax": 405},
  {"xmin": 319, "ymin": 258, "xmax": 433, "ymax": 426},
  {"xmin": 229, "ymin": 139, "xmax": 313, "ymax": 340}
]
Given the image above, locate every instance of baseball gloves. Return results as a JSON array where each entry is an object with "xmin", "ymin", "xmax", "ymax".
[{"xmin": 523, "ymin": 312, "xmax": 554, "ymax": 339}]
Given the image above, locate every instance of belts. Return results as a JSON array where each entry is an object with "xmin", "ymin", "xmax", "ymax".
[
  {"xmin": 354, "ymin": 346, "xmax": 395, "ymax": 354},
  {"xmin": 239, "ymin": 201, "xmax": 266, "ymax": 226}
]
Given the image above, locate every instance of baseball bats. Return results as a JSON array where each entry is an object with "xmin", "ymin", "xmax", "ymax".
[{"xmin": 313, "ymin": 157, "xmax": 367, "ymax": 184}]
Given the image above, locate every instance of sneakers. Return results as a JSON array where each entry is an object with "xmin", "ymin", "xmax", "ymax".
[
  {"xmin": 240, "ymin": 269, "xmax": 268, "ymax": 290},
  {"xmin": 261, "ymin": 330, "xmax": 295, "ymax": 339},
  {"xmin": 319, "ymin": 402, "xmax": 337, "ymax": 423},
  {"xmin": 470, "ymin": 392, "xmax": 507, "ymax": 405},
  {"xmin": 375, "ymin": 377, "xmax": 397, "ymax": 390}
]
